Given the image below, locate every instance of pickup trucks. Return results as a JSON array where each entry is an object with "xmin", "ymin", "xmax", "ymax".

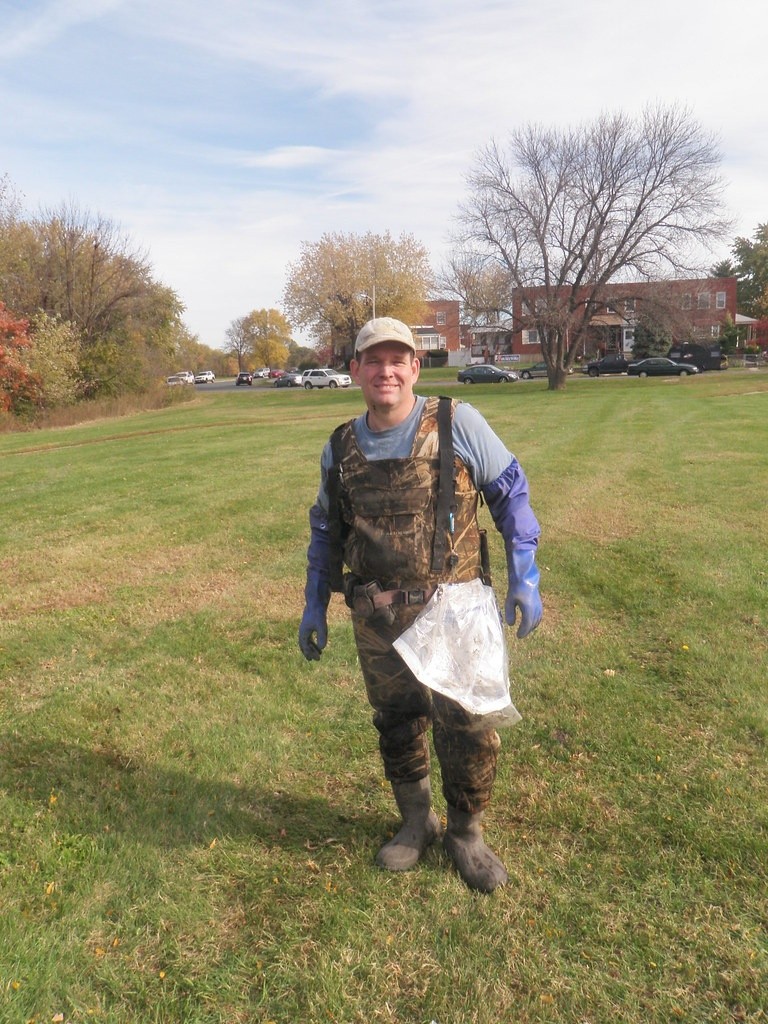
[{"xmin": 583, "ymin": 353, "xmax": 640, "ymax": 377}]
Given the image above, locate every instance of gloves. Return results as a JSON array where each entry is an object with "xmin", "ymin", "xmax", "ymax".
[
  {"xmin": 298, "ymin": 506, "xmax": 332, "ymax": 662},
  {"xmin": 480, "ymin": 457, "xmax": 543, "ymax": 638}
]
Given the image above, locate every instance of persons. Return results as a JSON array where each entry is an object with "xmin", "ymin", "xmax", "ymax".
[{"xmin": 299, "ymin": 317, "xmax": 543, "ymax": 894}]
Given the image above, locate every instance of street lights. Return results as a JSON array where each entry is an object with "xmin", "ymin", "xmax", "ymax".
[{"xmin": 360, "ymin": 294, "xmax": 376, "ymax": 318}]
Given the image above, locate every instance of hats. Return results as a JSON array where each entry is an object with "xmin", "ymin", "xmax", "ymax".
[{"xmin": 354, "ymin": 317, "xmax": 415, "ymax": 355}]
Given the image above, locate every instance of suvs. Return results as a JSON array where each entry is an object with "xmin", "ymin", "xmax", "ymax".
[
  {"xmin": 235, "ymin": 372, "xmax": 252, "ymax": 386},
  {"xmin": 301, "ymin": 368, "xmax": 352, "ymax": 389},
  {"xmin": 195, "ymin": 371, "xmax": 215, "ymax": 384},
  {"xmin": 253, "ymin": 368, "xmax": 271, "ymax": 378}
]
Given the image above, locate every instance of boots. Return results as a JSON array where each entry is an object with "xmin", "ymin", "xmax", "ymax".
[
  {"xmin": 377, "ymin": 775, "xmax": 440, "ymax": 871},
  {"xmin": 443, "ymin": 803, "xmax": 508, "ymax": 893}
]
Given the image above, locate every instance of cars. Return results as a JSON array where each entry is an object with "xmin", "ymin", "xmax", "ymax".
[
  {"xmin": 165, "ymin": 371, "xmax": 196, "ymax": 385},
  {"xmin": 274, "ymin": 374, "xmax": 301, "ymax": 387},
  {"xmin": 628, "ymin": 357, "xmax": 698, "ymax": 378},
  {"xmin": 519, "ymin": 361, "xmax": 574, "ymax": 379},
  {"xmin": 458, "ymin": 365, "xmax": 519, "ymax": 384},
  {"xmin": 269, "ymin": 370, "xmax": 285, "ymax": 378}
]
